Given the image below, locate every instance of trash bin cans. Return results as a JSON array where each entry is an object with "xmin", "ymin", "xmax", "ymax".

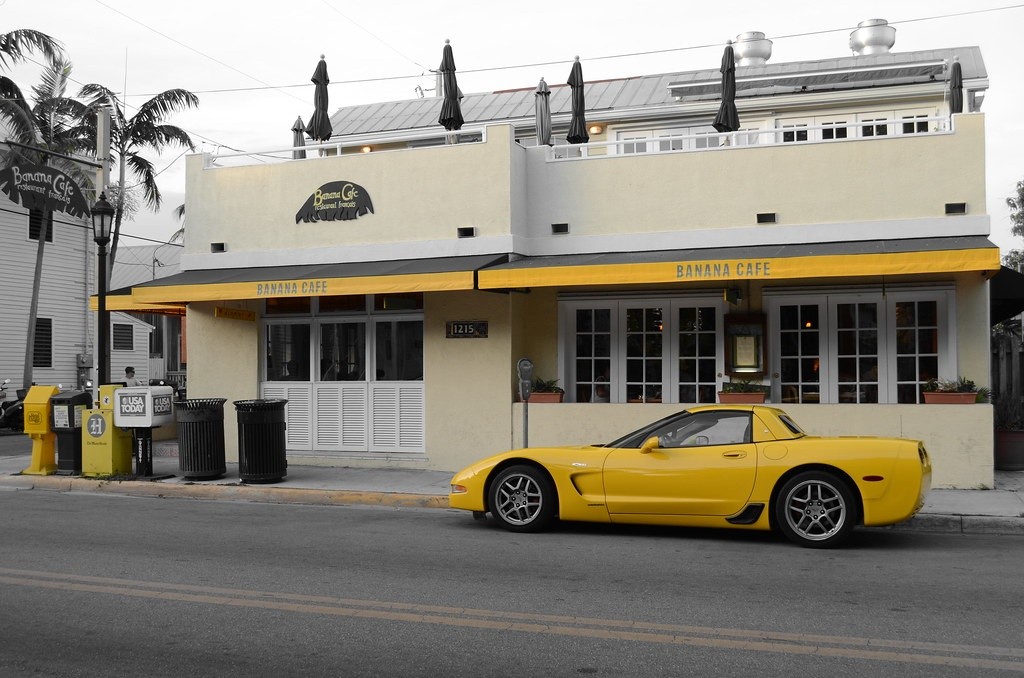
[
  {"xmin": 233, "ymin": 398, "xmax": 290, "ymax": 482},
  {"xmin": 175, "ymin": 397, "xmax": 227, "ymax": 480}
]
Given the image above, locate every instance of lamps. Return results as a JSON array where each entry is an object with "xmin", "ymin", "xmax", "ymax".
[
  {"xmin": 361, "ymin": 145, "xmax": 373, "ymax": 153},
  {"xmin": 590, "ymin": 125, "xmax": 604, "ymax": 135}
]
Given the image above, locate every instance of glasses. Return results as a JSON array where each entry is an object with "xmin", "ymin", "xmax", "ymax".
[{"xmin": 129, "ymin": 371, "xmax": 135, "ymax": 374}]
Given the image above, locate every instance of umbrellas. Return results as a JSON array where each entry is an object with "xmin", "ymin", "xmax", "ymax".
[
  {"xmin": 437, "ymin": 38, "xmax": 464, "ymax": 145},
  {"xmin": 305, "ymin": 54, "xmax": 333, "ymax": 158},
  {"xmin": 712, "ymin": 39, "xmax": 740, "ymax": 147},
  {"xmin": 566, "ymin": 56, "xmax": 590, "ymax": 156},
  {"xmin": 292, "ymin": 116, "xmax": 306, "ymax": 160},
  {"xmin": 534, "ymin": 77, "xmax": 552, "ymax": 146},
  {"xmin": 950, "ymin": 54, "xmax": 964, "ymax": 131}
]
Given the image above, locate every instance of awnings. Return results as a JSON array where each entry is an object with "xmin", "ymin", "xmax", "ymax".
[
  {"xmin": 478, "ymin": 238, "xmax": 1000, "ymax": 295},
  {"xmin": 990, "ymin": 265, "xmax": 1024, "ymax": 327},
  {"xmin": 90, "ymin": 254, "xmax": 509, "ymax": 317}
]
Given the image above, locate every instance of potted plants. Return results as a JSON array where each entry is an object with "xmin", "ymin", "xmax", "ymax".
[
  {"xmin": 715, "ymin": 375, "xmax": 769, "ymax": 404},
  {"xmin": 922, "ymin": 375, "xmax": 978, "ymax": 404},
  {"xmin": 518, "ymin": 376, "xmax": 566, "ymax": 403}
]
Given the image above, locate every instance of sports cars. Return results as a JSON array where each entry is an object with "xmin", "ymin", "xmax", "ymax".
[{"xmin": 448, "ymin": 404, "xmax": 932, "ymax": 549}]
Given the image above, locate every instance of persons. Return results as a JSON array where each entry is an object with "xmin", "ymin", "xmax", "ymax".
[
  {"xmin": 121, "ymin": 367, "xmax": 143, "ymax": 387},
  {"xmin": 589, "ymin": 362, "xmax": 610, "ymax": 403},
  {"xmin": 282, "ymin": 360, "xmax": 305, "ymax": 381},
  {"xmin": 680, "ymin": 353, "xmax": 696, "ymax": 403}
]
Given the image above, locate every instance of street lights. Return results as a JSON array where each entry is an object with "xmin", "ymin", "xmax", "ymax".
[{"xmin": 90, "ymin": 190, "xmax": 114, "ymax": 408}]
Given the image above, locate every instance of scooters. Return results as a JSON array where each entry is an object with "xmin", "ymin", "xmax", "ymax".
[{"xmin": 0, "ymin": 379, "xmax": 29, "ymax": 436}]
